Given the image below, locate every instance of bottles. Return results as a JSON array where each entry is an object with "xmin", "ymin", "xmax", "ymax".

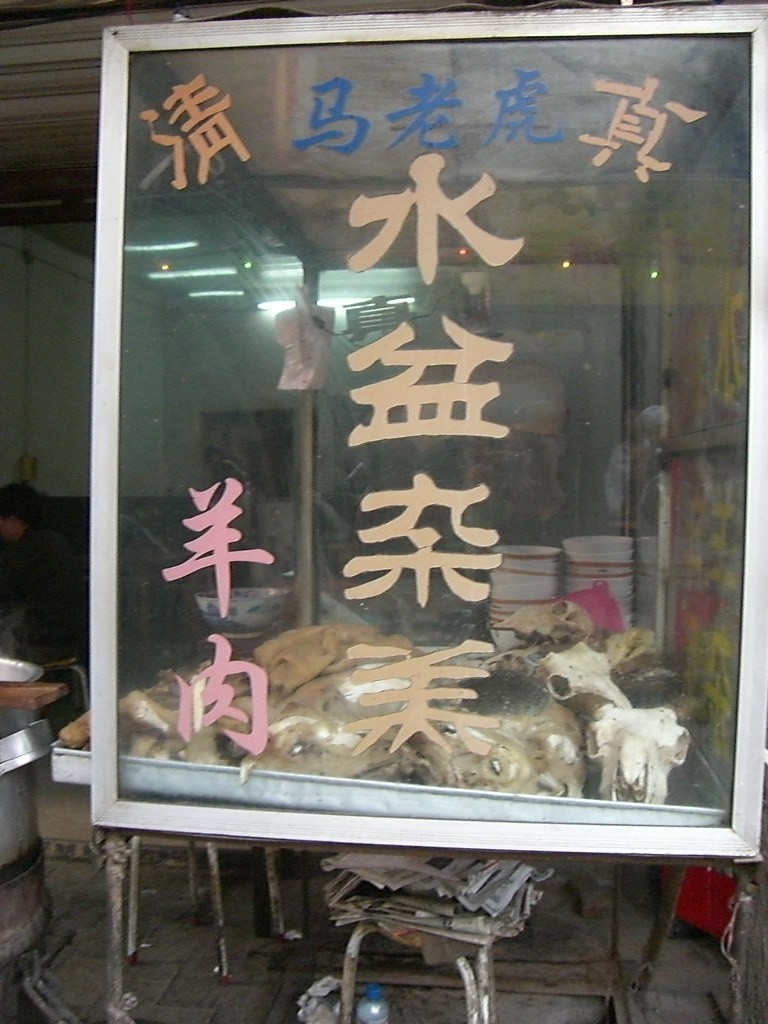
[{"xmin": 354, "ymin": 983, "xmax": 390, "ymax": 1024}]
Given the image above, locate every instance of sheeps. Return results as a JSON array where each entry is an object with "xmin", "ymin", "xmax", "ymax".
[{"xmin": 57, "ymin": 596, "xmax": 694, "ymax": 808}]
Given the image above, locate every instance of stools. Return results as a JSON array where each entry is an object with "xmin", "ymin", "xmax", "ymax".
[{"xmin": 336, "ymin": 923, "xmax": 509, "ymax": 1024}]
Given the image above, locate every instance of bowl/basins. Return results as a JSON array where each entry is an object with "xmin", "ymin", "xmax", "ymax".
[
  {"xmin": 490, "ymin": 533, "xmax": 638, "ymax": 651},
  {"xmin": 195, "ymin": 586, "xmax": 292, "ymax": 641}
]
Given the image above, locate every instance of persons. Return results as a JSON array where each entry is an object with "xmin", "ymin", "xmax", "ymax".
[{"xmin": 0, "ymin": 483, "xmax": 80, "ymax": 665}]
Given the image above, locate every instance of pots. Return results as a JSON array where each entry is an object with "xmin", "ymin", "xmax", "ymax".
[{"xmin": 0, "ymin": 720, "xmax": 57, "ymax": 887}]
